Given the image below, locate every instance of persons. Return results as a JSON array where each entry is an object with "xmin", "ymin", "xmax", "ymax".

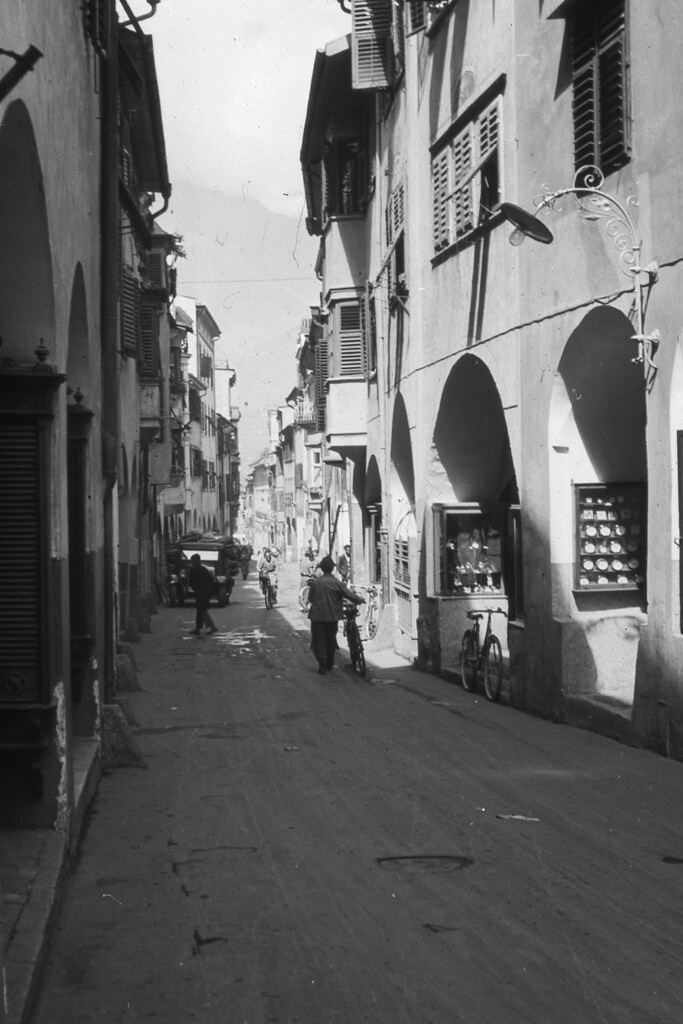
[
  {"xmin": 309, "ymin": 557, "xmax": 366, "ymax": 674},
  {"xmin": 188, "ymin": 554, "xmax": 218, "ymax": 634},
  {"xmin": 256, "ymin": 547, "xmax": 278, "ymax": 598},
  {"xmin": 337, "ymin": 545, "xmax": 350, "ymax": 585},
  {"xmin": 239, "ymin": 548, "xmax": 250, "ymax": 580},
  {"xmin": 298, "ymin": 551, "xmax": 322, "ymax": 610}
]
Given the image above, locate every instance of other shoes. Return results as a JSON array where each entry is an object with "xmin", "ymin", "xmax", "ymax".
[
  {"xmin": 189, "ymin": 629, "xmax": 200, "ymax": 635},
  {"xmin": 327, "ymin": 666, "xmax": 332, "ymax": 671},
  {"xmin": 318, "ymin": 661, "xmax": 327, "ymax": 675},
  {"xmin": 206, "ymin": 627, "xmax": 218, "ymax": 634}
]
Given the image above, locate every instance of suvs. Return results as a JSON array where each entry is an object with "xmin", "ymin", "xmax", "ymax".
[{"xmin": 171, "ymin": 542, "xmax": 235, "ymax": 609}]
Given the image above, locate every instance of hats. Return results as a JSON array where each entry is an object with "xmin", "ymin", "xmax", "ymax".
[{"xmin": 316, "ymin": 556, "xmax": 337, "ymax": 568}]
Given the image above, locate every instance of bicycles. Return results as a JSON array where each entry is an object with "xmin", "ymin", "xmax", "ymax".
[
  {"xmin": 343, "ymin": 585, "xmax": 381, "ymax": 639},
  {"xmin": 259, "ymin": 568, "xmax": 279, "ymax": 610},
  {"xmin": 297, "ymin": 572, "xmax": 319, "ymax": 613},
  {"xmin": 458, "ymin": 608, "xmax": 509, "ymax": 704},
  {"xmin": 343, "ymin": 599, "xmax": 368, "ymax": 679}
]
